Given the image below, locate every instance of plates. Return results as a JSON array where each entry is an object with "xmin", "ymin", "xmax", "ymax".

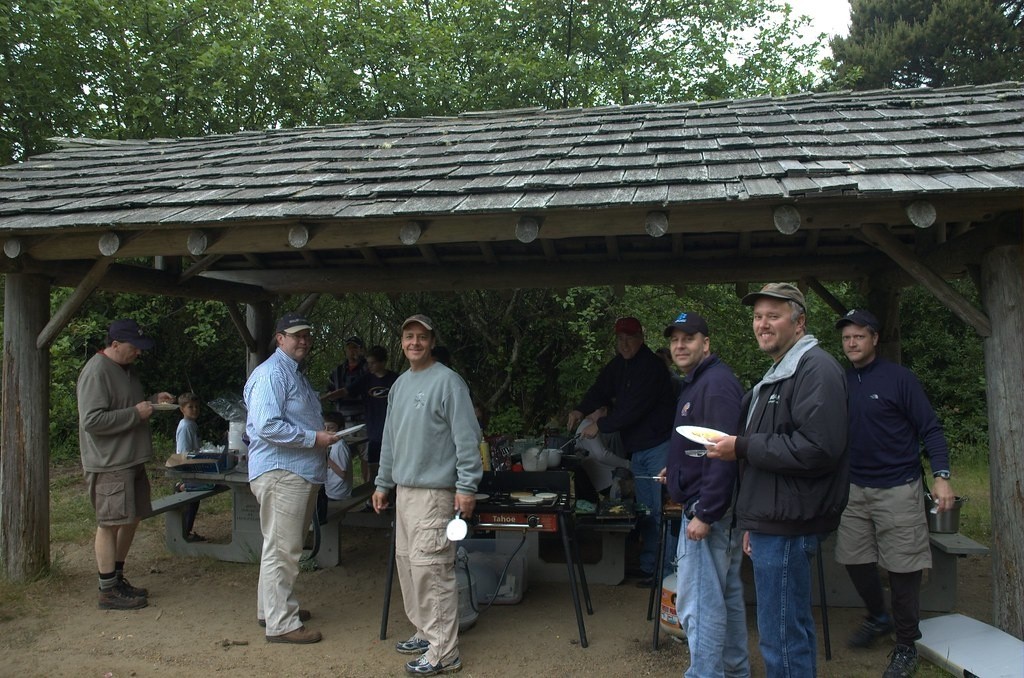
[
  {"xmin": 676, "ymin": 425, "xmax": 732, "ymax": 446},
  {"xmin": 334, "ymin": 424, "xmax": 367, "ymax": 436},
  {"xmin": 149, "ymin": 404, "xmax": 181, "ymax": 410}
]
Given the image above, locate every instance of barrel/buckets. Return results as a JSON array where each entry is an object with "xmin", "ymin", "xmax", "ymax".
[{"xmin": 926, "ymin": 494, "xmax": 963, "ymax": 534}]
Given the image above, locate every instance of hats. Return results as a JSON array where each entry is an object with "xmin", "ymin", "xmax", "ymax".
[
  {"xmin": 615, "ymin": 317, "xmax": 642, "ymax": 336},
  {"xmin": 663, "ymin": 312, "xmax": 708, "ymax": 337},
  {"xmin": 835, "ymin": 308, "xmax": 882, "ymax": 332},
  {"xmin": 345, "ymin": 336, "xmax": 362, "ymax": 346},
  {"xmin": 109, "ymin": 319, "xmax": 154, "ymax": 349},
  {"xmin": 275, "ymin": 312, "xmax": 313, "ymax": 334},
  {"xmin": 742, "ymin": 282, "xmax": 808, "ymax": 314},
  {"xmin": 402, "ymin": 314, "xmax": 435, "ymax": 331}
]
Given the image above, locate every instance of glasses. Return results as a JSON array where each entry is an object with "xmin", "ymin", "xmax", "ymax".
[{"xmin": 285, "ymin": 333, "xmax": 314, "ymax": 339}]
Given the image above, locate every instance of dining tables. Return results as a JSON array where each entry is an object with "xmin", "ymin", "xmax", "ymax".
[{"xmin": 177, "ymin": 435, "xmax": 370, "ymax": 564}]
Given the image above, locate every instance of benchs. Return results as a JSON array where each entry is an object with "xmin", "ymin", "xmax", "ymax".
[
  {"xmin": 141, "ymin": 483, "xmax": 230, "ymax": 560},
  {"xmin": 299, "ymin": 478, "xmax": 377, "ymax": 567},
  {"xmin": 921, "ymin": 530, "xmax": 990, "ymax": 612}
]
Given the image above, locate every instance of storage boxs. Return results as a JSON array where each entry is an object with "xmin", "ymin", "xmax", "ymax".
[
  {"xmin": 229, "ymin": 423, "xmax": 249, "ymax": 474},
  {"xmin": 165, "ymin": 446, "xmax": 239, "ymax": 471}
]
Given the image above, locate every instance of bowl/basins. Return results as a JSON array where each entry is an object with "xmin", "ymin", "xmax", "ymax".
[
  {"xmin": 521, "ymin": 447, "xmax": 550, "ymax": 471},
  {"xmin": 544, "ymin": 449, "xmax": 564, "ymax": 468}
]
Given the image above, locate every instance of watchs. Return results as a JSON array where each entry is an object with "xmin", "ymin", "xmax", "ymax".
[
  {"xmin": 370, "ymin": 312, "xmax": 487, "ymax": 678},
  {"xmin": 933, "ymin": 471, "xmax": 950, "ymax": 480}
]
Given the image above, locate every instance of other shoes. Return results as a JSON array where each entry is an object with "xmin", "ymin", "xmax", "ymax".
[
  {"xmin": 625, "ymin": 563, "xmax": 651, "ymax": 579},
  {"xmin": 636, "ymin": 573, "xmax": 659, "ymax": 588},
  {"xmin": 186, "ymin": 531, "xmax": 206, "ymax": 543}
]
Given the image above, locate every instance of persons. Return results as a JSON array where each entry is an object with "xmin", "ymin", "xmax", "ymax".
[
  {"xmin": 814, "ymin": 308, "xmax": 956, "ymax": 678},
  {"xmin": 311, "ymin": 346, "xmax": 397, "ymax": 514},
  {"xmin": 172, "ymin": 390, "xmax": 206, "ymax": 545},
  {"xmin": 237, "ymin": 311, "xmax": 341, "ymax": 648},
  {"xmin": 324, "ymin": 337, "xmax": 374, "ymax": 485},
  {"xmin": 704, "ymin": 282, "xmax": 853, "ymax": 678},
  {"xmin": 565, "ymin": 315, "xmax": 685, "ymax": 592},
  {"xmin": 74, "ymin": 319, "xmax": 179, "ymax": 612},
  {"xmin": 653, "ymin": 346, "xmax": 684, "ymax": 398},
  {"xmin": 574, "ymin": 395, "xmax": 634, "ymax": 500},
  {"xmin": 431, "ymin": 346, "xmax": 489, "ymax": 430},
  {"xmin": 302, "ymin": 410, "xmax": 359, "ymax": 550},
  {"xmin": 652, "ymin": 312, "xmax": 758, "ymax": 677}
]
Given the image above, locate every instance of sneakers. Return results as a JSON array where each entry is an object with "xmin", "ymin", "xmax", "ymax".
[
  {"xmin": 881, "ymin": 644, "xmax": 919, "ymax": 678},
  {"xmin": 395, "ymin": 636, "xmax": 430, "ymax": 655},
  {"xmin": 405, "ymin": 654, "xmax": 463, "ymax": 678},
  {"xmin": 266, "ymin": 626, "xmax": 322, "ymax": 644},
  {"xmin": 848, "ymin": 615, "xmax": 893, "ymax": 648},
  {"xmin": 97, "ymin": 585, "xmax": 148, "ymax": 610},
  {"xmin": 116, "ymin": 574, "xmax": 148, "ymax": 597},
  {"xmin": 258, "ymin": 609, "xmax": 311, "ymax": 627}
]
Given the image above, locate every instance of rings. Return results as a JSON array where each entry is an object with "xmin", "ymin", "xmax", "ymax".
[{"xmin": 696, "ymin": 537, "xmax": 702, "ymax": 540}]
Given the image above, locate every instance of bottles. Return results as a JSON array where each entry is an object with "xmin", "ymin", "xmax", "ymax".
[{"xmin": 478, "ymin": 436, "xmax": 491, "ymax": 472}]
